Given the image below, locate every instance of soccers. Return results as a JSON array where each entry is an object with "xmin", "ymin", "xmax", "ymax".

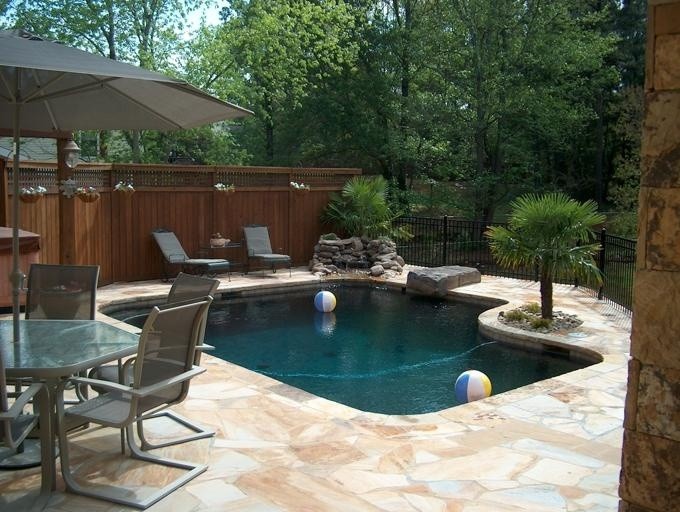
[
  {"xmin": 454, "ymin": 369, "xmax": 491, "ymax": 403},
  {"xmin": 314, "ymin": 290, "xmax": 336, "ymax": 313}
]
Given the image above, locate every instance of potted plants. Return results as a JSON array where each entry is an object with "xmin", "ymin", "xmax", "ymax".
[
  {"xmin": 75, "ymin": 185, "xmax": 100, "ymax": 202},
  {"xmin": 213, "ymin": 182, "xmax": 236, "ymax": 195},
  {"xmin": 287, "ymin": 181, "xmax": 311, "ymax": 196},
  {"xmin": 18, "ymin": 185, "xmax": 46, "ymax": 203},
  {"xmin": 114, "ymin": 180, "xmax": 136, "ymax": 196}
]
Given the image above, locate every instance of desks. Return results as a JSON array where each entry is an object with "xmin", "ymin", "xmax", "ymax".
[
  {"xmin": 198, "ymin": 241, "xmax": 244, "ymax": 274},
  {"xmin": 0, "ymin": 319, "xmax": 149, "ymax": 469}
]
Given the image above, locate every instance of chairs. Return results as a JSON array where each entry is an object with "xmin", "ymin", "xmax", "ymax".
[
  {"xmin": 89, "ymin": 271, "xmax": 221, "ymax": 452},
  {"xmin": 7, "ymin": 263, "xmax": 100, "ymax": 437},
  {"xmin": 240, "ymin": 223, "xmax": 291, "ymax": 279},
  {"xmin": 57, "ymin": 294, "xmax": 213, "ymax": 509},
  {"xmin": 151, "ymin": 228, "xmax": 231, "ymax": 283},
  {"xmin": 1, "ymin": 382, "xmax": 53, "ymax": 512}
]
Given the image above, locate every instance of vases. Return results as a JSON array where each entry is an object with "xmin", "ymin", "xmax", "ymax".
[{"xmin": 37, "ymin": 286, "xmax": 83, "ymax": 319}]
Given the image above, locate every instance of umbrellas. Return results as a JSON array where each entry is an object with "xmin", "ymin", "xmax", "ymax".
[{"xmin": 0, "ymin": 26, "xmax": 256, "ymax": 465}]
